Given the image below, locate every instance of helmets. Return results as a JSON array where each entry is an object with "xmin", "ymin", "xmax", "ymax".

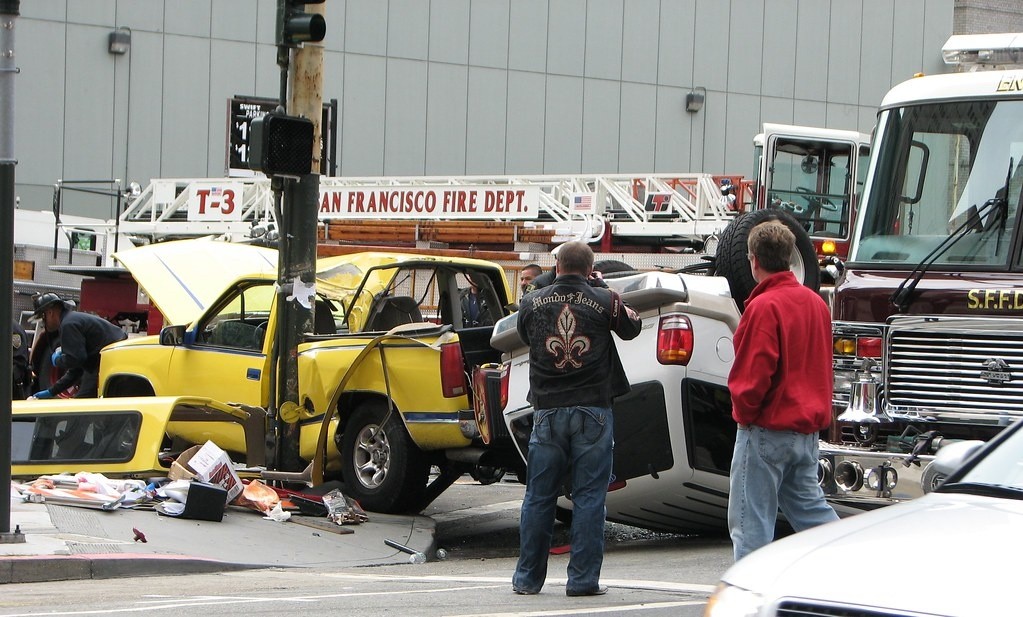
[{"xmin": 31, "ymin": 293, "xmax": 62, "ymax": 318}]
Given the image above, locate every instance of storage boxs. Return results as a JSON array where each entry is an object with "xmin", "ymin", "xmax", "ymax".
[{"xmin": 167, "ymin": 439, "xmax": 245, "ymax": 508}]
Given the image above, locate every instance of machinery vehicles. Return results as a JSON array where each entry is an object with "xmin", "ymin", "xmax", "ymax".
[{"xmin": 13, "ymin": 122, "xmax": 902, "ymax": 333}]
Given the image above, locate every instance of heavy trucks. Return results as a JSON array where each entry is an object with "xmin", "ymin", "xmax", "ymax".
[{"xmin": 817, "ymin": 30, "xmax": 1023, "ymax": 507}]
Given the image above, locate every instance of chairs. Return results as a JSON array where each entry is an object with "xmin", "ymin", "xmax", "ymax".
[
  {"xmin": 314, "ymin": 300, "xmax": 337, "ymax": 336},
  {"xmin": 371, "ymin": 297, "xmax": 423, "ymax": 333}
]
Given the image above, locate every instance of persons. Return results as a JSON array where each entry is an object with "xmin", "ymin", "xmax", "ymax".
[
  {"xmin": 511, "ymin": 243, "xmax": 641, "ymax": 595},
  {"xmin": 457, "ymin": 275, "xmax": 495, "ymax": 327},
  {"xmin": 518, "ymin": 240, "xmax": 582, "ymax": 296},
  {"xmin": 727, "ymin": 217, "xmax": 848, "ymax": 563},
  {"xmin": 12, "ymin": 292, "xmax": 128, "ymax": 460}
]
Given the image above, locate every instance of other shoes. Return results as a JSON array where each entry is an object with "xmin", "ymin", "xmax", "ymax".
[
  {"xmin": 567, "ymin": 586, "xmax": 608, "ymax": 596},
  {"xmin": 513, "ymin": 587, "xmax": 534, "ymax": 594}
]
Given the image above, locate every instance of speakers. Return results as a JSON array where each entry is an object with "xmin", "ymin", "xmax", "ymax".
[{"xmin": 248, "ymin": 114, "xmax": 314, "ymax": 176}]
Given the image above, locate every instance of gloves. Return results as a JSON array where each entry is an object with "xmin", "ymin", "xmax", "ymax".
[
  {"xmin": 52, "ymin": 347, "xmax": 62, "ymax": 366},
  {"xmin": 33, "ymin": 389, "xmax": 54, "ymax": 399}
]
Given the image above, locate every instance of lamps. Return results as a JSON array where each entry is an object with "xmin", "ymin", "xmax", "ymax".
[
  {"xmin": 686, "ymin": 92, "xmax": 705, "ymax": 112},
  {"xmin": 108, "ymin": 31, "xmax": 130, "ymax": 55}
]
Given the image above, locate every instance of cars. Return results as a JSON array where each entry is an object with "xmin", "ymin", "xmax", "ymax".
[
  {"xmin": 705, "ymin": 421, "xmax": 1023, "ymax": 617},
  {"xmin": 489, "ymin": 209, "xmax": 819, "ymax": 536},
  {"xmin": 95, "ymin": 240, "xmax": 513, "ymax": 515}
]
